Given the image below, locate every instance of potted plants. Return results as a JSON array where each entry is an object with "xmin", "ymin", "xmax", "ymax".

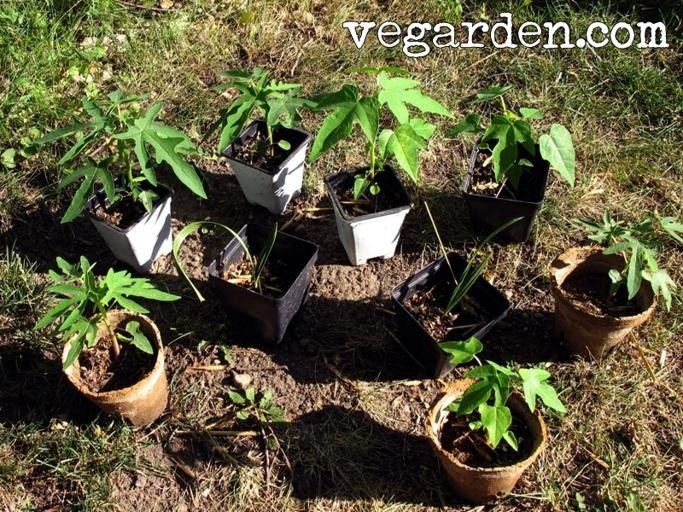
[{"xmin": 33, "ymin": 63, "xmax": 675, "ymax": 500}]
